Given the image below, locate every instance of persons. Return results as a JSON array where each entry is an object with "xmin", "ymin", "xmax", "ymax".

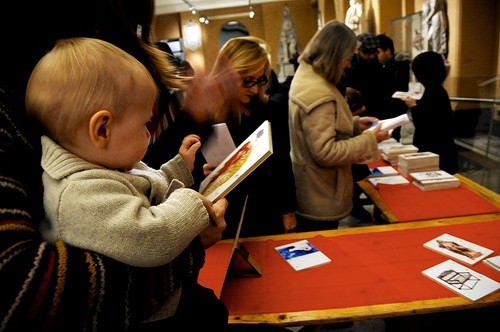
[
  {"xmin": 402, "ymin": 51, "xmax": 459, "ymax": 175},
  {"xmin": 422, "ymin": 0, "xmax": 449, "ymax": 65},
  {"xmin": 0, "ymin": 0, "xmax": 227, "ymax": 332},
  {"xmin": 25, "ymin": 37, "xmax": 228, "ymax": 331},
  {"xmin": 151, "ymin": 0, "xmax": 412, "ymax": 131},
  {"xmin": 287, "ymin": 21, "xmax": 389, "ymax": 231},
  {"xmin": 202, "ymin": 37, "xmax": 297, "ymax": 239}
]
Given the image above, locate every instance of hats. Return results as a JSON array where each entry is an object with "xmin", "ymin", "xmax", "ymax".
[{"xmin": 357, "ymin": 32, "xmax": 379, "ymax": 49}]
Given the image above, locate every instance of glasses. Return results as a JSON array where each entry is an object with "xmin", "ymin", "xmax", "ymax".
[
  {"xmin": 362, "ymin": 48, "xmax": 379, "ymax": 56},
  {"xmin": 241, "ymin": 74, "xmax": 268, "ymax": 88}
]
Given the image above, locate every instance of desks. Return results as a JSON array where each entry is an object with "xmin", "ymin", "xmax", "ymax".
[
  {"xmin": 357, "ymin": 154, "xmax": 500, "ymax": 224},
  {"xmin": 196, "ymin": 212, "xmax": 499, "ymax": 324}
]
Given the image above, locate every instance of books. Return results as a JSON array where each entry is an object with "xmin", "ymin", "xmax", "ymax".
[
  {"xmin": 198, "ymin": 123, "xmax": 236, "ymax": 170},
  {"xmin": 198, "ymin": 121, "xmax": 273, "ymax": 202},
  {"xmin": 274, "ymin": 238, "xmax": 333, "ymax": 272},
  {"xmin": 360, "ymin": 91, "xmax": 500, "ymax": 304}
]
